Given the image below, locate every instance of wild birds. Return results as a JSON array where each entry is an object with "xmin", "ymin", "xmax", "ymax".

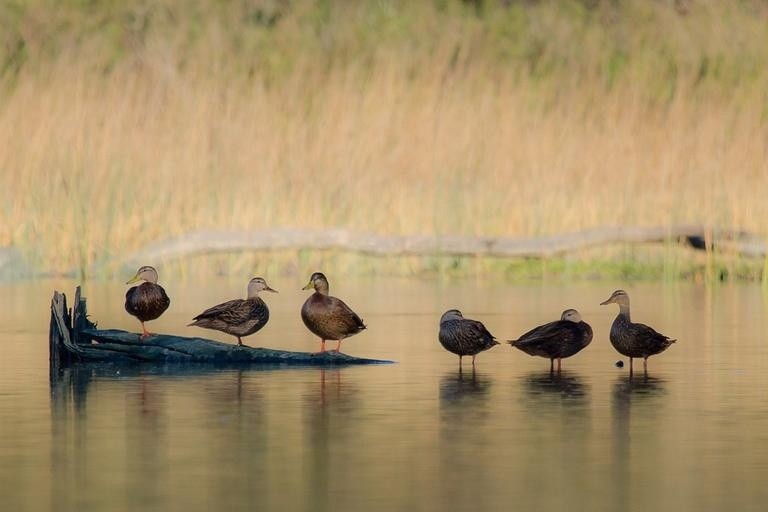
[
  {"xmin": 504, "ymin": 309, "xmax": 592, "ymax": 369},
  {"xmin": 300, "ymin": 271, "xmax": 366, "ymax": 354},
  {"xmin": 122, "ymin": 265, "xmax": 170, "ymax": 340},
  {"xmin": 600, "ymin": 290, "xmax": 677, "ymax": 369},
  {"xmin": 187, "ymin": 276, "xmax": 280, "ymax": 346},
  {"xmin": 438, "ymin": 308, "xmax": 501, "ymax": 367}
]
